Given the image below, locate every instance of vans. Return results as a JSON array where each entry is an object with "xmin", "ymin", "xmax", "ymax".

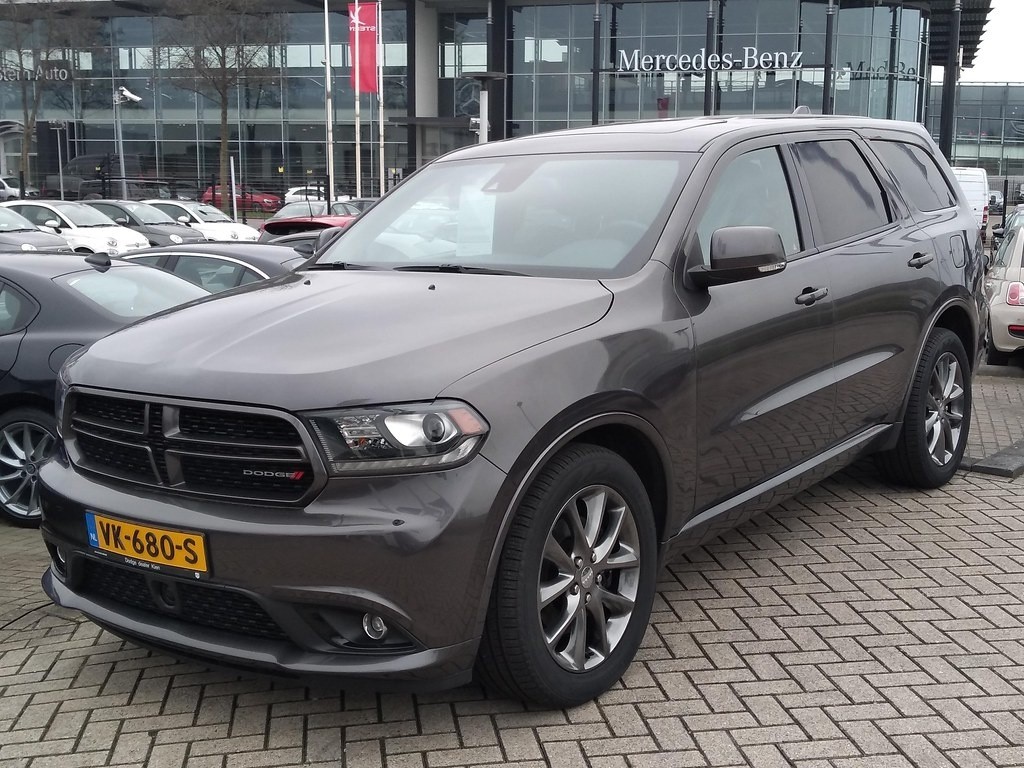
[{"xmin": 46, "ymin": 154, "xmax": 176, "ymax": 200}]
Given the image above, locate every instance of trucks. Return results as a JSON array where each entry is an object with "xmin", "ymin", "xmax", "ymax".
[{"xmin": 949, "ymin": 166, "xmax": 990, "ymax": 243}]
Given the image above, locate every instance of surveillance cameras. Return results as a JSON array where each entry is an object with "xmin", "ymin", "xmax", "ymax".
[{"xmin": 118, "ymin": 86, "xmax": 142, "ymax": 103}]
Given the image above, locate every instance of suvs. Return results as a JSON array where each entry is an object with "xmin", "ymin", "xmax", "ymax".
[{"xmin": 37, "ymin": 107, "xmax": 988, "ymax": 710}]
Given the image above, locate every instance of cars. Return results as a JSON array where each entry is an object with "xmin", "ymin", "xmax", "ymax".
[
  {"xmin": 983, "ymin": 204, "xmax": 1024, "ymax": 365},
  {"xmin": 1012, "ymin": 183, "xmax": 1024, "ymax": 204},
  {"xmin": 0, "ymin": 175, "xmax": 41, "ymax": 201},
  {"xmin": 285, "ymin": 186, "xmax": 357, "ymax": 206},
  {"xmin": 201, "ymin": 184, "xmax": 282, "ymax": 213},
  {"xmin": 990, "ymin": 191, "xmax": 1004, "ymax": 214},
  {"xmin": 0, "ymin": 199, "xmax": 458, "ymax": 527}
]
